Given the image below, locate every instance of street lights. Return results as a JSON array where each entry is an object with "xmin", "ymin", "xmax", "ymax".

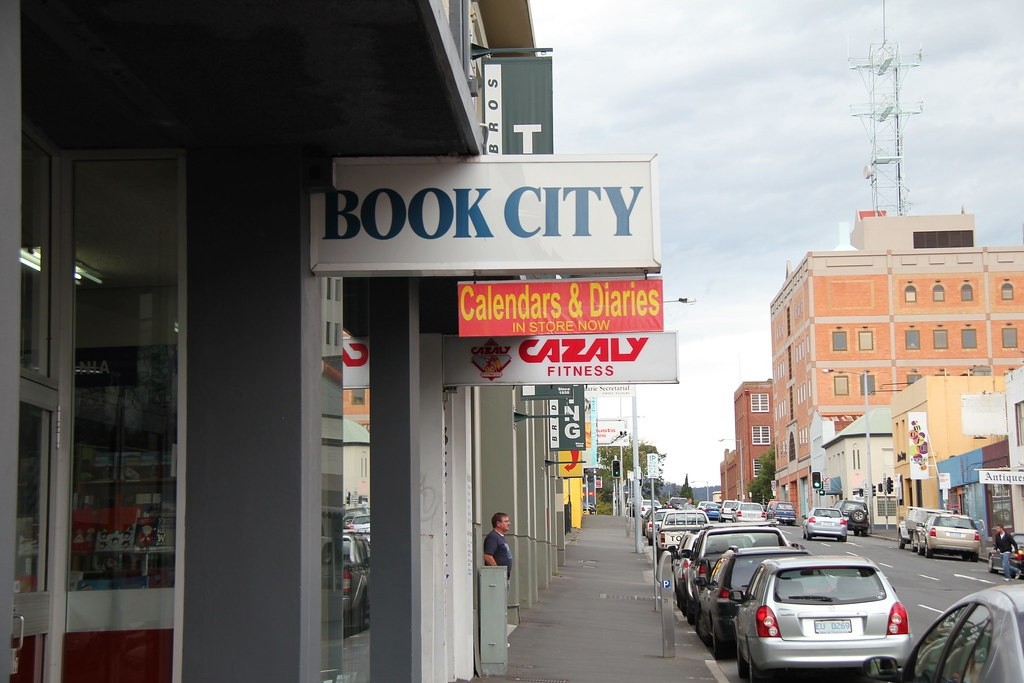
[
  {"xmin": 822, "ymin": 368, "xmax": 875, "ymax": 535},
  {"xmin": 632, "ymin": 299, "xmax": 698, "ymax": 552},
  {"xmin": 691, "ymin": 481, "xmax": 709, "ymax": 501},
  {"xmin": 719, "ymin": 438, "xmax": 744, "ymax": 502}
]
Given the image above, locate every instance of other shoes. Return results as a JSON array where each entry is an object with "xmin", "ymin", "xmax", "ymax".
[{"xmin": 507, "ymin": 642, "xmax": 511, "ymax": 648}]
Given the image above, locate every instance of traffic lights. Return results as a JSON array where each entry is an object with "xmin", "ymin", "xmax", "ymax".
[
  {"xmin": 888, "ymin": 477, "xmax": 893, "ymax": 494},
  {"xmin": 612, "ymin": 460, "xmax": 620, "ymax": 478},
  {"xmin": 812, "ymin": 472, "xmax": 822, "ymax": 490},
  {"xmin": 820, "ymin": 490, "xmax": 825, "ymax": 495},
  {"xmin": 859, "ymin": 488, "xmax": 863, "ymax": 497},
  {"xmin": 872, "ymin": 485, "xmax": 877, "ymax": 497}
]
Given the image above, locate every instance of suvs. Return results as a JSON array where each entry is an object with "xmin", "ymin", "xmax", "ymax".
[
  {"xmin": 667, "ymin": 528, "xmax": 792, "ymax": 625},
  {"xmin": 831, "ymin": 500, "xmax": 869, "ymax": 537},
  {"xmin": 897, "ymin": 507, "xmax": 947, "ymax": 551},
  {"xmin": 917, "ymin": 512, "xmax": 980, "ymax": 562},
  {"xmin": 693, "ymin": 546, "xmax": 833, "ymax": 659},
  {"xmin": 761, "ymin": 501, "xmax": 796, "ymax": 525}
]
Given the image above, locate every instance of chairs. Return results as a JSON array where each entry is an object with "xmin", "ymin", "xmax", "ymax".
[
  {"xmin": 779, "ymin": 581, "xmax": 805, "ymax": 600},
  {"xmin": 655, "ymin": 513, "xmax": 662, "ymax": 520},
  {"xmin": 753, "ymin": 538, "xmax": 779, "ymax": 546},
  {"xmin": 691, "ymin": 517, "xmax": 701, "ymax": 524},
  {"xmin": 831, "ymin": 512, "xmax": 836, "ymax": 517},
  {"xmin": 737, "ymin": 536, "xmax": 752, "ymax": 547},
  {"xmin": 712, "ymin": 539, "xmax": 729, "ymax": 552},
  {"xmin": 670, "ymin": 517, "xmax": 678, "ymax": 523},
  {"xmin": 816, "ymin": 511, "xmax": 822, "ymax": 516},
  {"xmin": 836, "ymin": 576, "xmax": 858, "ymax": 597}
]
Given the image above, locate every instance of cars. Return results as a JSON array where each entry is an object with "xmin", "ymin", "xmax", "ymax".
[
  {"xmin": 731, "ymin": 502, "xmax": 767, "ymax": 523},
  {"xmin": 862, "ymin": 579, "xmax": 1024, "ymax": 683},
  {"xmin": 987, "ymin": 532, "xmax": 1024, "ymax": 575},
  {"xmin": 727, "ymin": 556, "xmax": 915, "ymax": 683},
  {"xmin": 717, "ymin": 500, "xmax": 739, "ymax": 522},
  {"xmin": 628, "ymin": 497, "xmax": 688, "ymax": 546},
  {"xmin": 801, "ymin": 507, "xmax": 847, "ymax": 542},
  {"xmin": 342, "ymin": 506, "xmax": 370, "ymax": 634}
]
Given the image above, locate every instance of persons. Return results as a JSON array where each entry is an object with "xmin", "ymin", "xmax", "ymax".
[
  {"xmin": 484, "ymin": 512, "xmax": 513, "ymax": 647},
  {"xmin": 992, "ymin": 524, "xmax": 1022, "ymax": 581},
  {"xmin": 684, "ymin": 498, "xmax": 693, "ymax": 510}
]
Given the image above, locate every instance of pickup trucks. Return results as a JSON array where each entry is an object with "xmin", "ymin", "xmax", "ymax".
[{"xmin": 656, "ymin": 510, "xmax": 713, "ymax": 563}]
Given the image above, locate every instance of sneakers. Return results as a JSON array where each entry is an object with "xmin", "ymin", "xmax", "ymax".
[
  {"xmin": 1015, "ymin": 570, "xmax": 1021, "ymax": 579},
  {"xmin": 1003, "ymin": 578, "xmax": 1011, "ymax": 582}
]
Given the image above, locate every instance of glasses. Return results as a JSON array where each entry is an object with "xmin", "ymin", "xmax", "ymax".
[{"xmin": 503, "ymin": 520, "xmax": 510, "ymax": 523}]
